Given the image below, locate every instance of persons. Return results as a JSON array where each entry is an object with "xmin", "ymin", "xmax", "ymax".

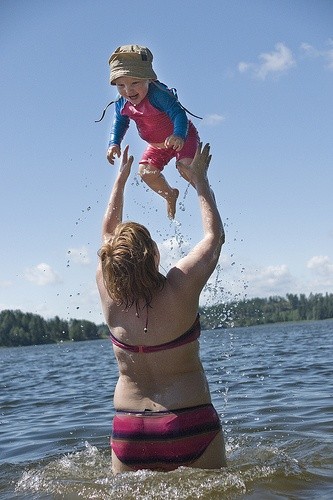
[
  {"xmin": 94, "ymin": 44, "xmax": 223, "ymax": 221},
  {"xmin": 96, "ymin": 142, "xmax": 229, "ymax": 473}
]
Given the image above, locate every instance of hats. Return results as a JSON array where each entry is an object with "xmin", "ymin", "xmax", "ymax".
[{"xmin": 109, "ymin": 44, "xmax": 158, "ymax": 85}]
